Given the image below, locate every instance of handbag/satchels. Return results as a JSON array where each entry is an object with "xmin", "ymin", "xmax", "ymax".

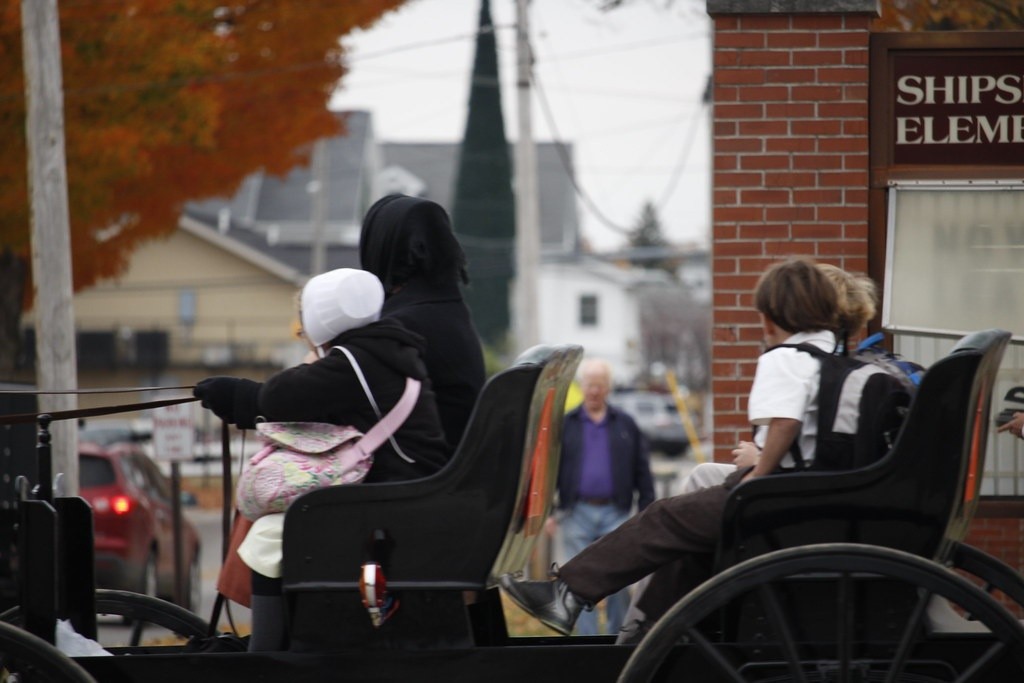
[{"xmin": 234, "ymin": 421, "xmax": 375, "ymax": 522}]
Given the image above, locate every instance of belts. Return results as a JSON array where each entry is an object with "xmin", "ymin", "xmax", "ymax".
[{"xmin": 577, "ymin": 494, "xmax": 617, "ymax": 508}]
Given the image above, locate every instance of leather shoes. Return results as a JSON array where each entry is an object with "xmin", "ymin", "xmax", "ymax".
[{"xmin": 501, "ymin": 571, "xmax": 587, "ymax": 636}]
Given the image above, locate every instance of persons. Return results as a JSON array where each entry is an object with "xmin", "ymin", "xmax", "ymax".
[
  {"xmin": 612, "ymin": 265, "xmax": 879, "ymax": 645},
  {"xmin": 192, "ymin": 191, "xmax": 487, "ymax": 484},
  {"xmin": 498, "ymin": 258, "xmax": 844, "ymax": 637},
  {"xmin": 547, "ymin": 358, "xmax": 657, "ymax": 636},
  {"xmin": 244, "ymin": 267, "xmax": 450, "ymax": 657}
]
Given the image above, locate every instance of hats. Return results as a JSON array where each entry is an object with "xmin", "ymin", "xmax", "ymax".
[{"xmin": 301, "ymin": 267, "xmax": 384, "ymax": 347}]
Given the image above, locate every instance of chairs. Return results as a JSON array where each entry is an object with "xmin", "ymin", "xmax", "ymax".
[{"xmin": 278, "ymin": 324, "xmax": 1016, "ymax": 650}]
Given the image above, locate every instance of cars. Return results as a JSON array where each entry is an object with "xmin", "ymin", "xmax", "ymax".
[{"xmin": 73, "ymin": 420, "xmax": 202, "ymax": 619}]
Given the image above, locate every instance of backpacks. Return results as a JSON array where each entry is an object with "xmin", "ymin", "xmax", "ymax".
[{"xmin": 746, "ymin": 339, "xmax": 928, "ymax": 478}]
